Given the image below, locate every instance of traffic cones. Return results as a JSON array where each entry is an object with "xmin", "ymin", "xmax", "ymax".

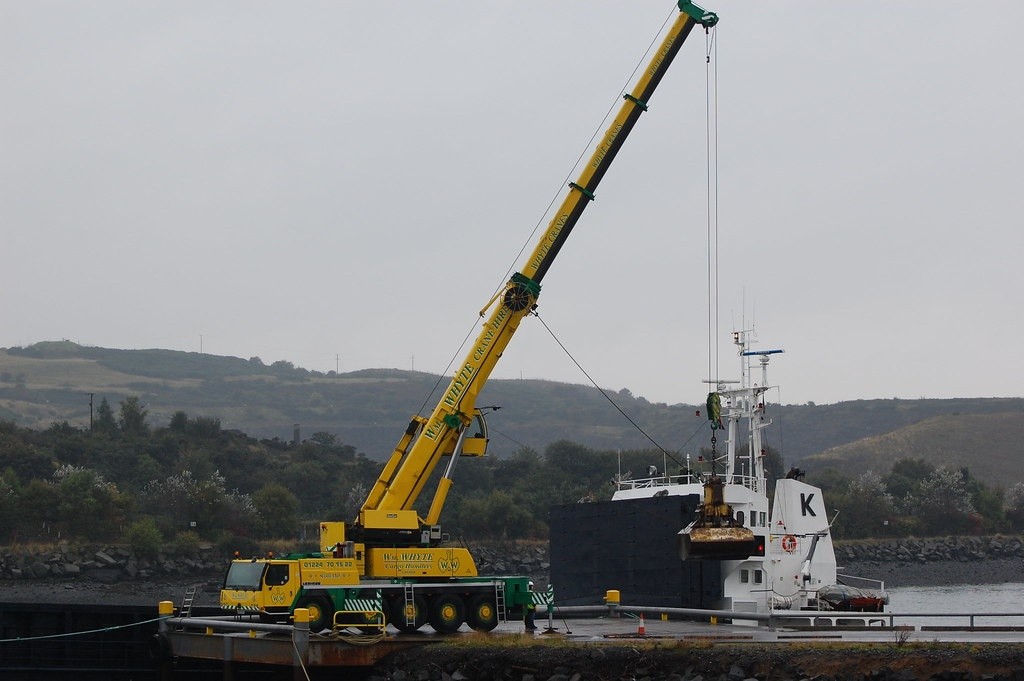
[{"xmin": 636, "ymin": 611, "xmax": 649, "ymax": 635}]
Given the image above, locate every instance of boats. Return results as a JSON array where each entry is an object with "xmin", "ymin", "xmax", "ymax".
[
  {"xmin": 816, "ymin": 586, "xmax": 886, "ymax": 613},
  {"xmin": 611, "ymin": 281, "xmax": 890, "ymax": 628}
]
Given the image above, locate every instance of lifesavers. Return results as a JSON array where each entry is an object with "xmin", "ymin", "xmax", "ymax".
[{"xmin": 782, "ymin": 533, "xmax": 797, "ymax": 552}]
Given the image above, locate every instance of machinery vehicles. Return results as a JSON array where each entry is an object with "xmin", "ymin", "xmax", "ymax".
[{"xmin": 218, "ymin": 0, "xmax": 720, "ymax": 636}]
